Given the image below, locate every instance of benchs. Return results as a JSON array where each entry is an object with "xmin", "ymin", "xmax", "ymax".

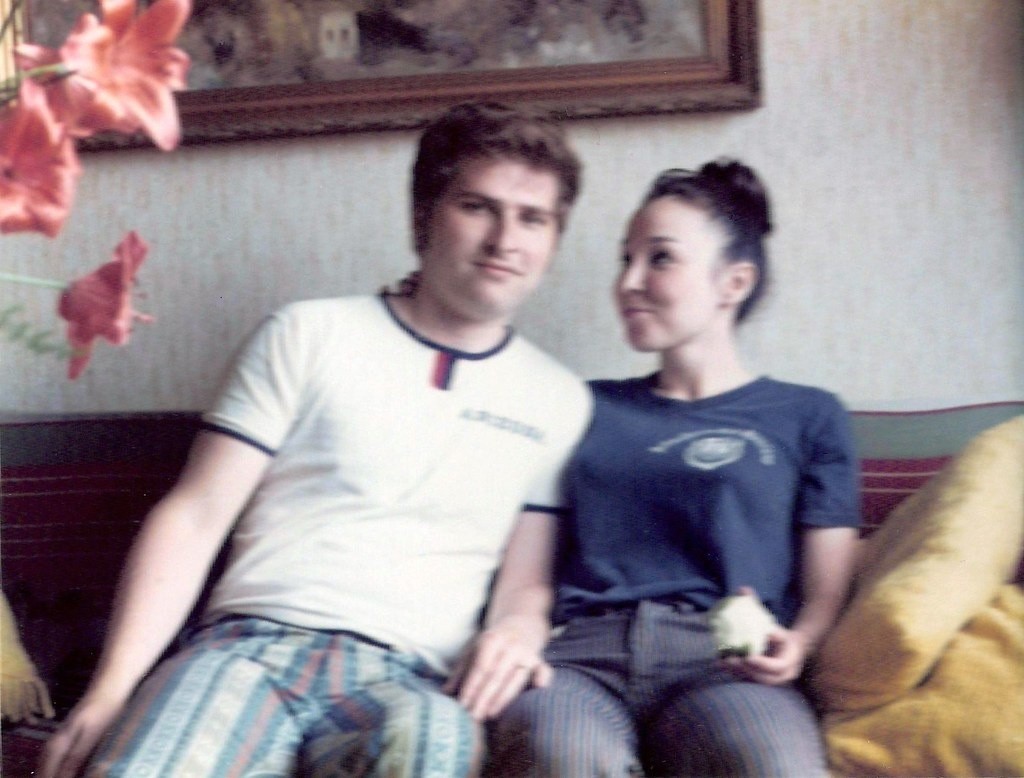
[{"xmin": 0, "ymin": 401, "xmax": 1024, "ymax": 778}]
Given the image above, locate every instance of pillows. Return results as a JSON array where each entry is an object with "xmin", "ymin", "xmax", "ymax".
[{"xmin": 803, "ymin": 414, "xmax": 1024, "ymax": 717}]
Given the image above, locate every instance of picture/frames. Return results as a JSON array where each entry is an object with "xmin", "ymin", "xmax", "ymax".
[{"xmin": 25, "ymin": 0, "xmax": 762, "ymax": 161}]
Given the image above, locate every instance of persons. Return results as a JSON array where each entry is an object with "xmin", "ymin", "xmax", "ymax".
[
  {"xmin": 0, "ymin": 590, "xmax": 55, "ymax": 724},
  {"xmin": 486, "ymin": 157, "xmax": 861, "ymax": 778},
  {"xmin": 39, "ymin": 101, "xmax": 597, "ymax": 778}
]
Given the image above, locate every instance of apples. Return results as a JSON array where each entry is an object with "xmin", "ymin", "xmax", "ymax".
[{"xmin": 706, "ymin": 594, "xmax": 773, "ymax": 658}]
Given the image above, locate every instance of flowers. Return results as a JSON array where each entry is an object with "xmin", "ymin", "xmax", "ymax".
[{"xmin": 0, "ymin": 0, "xmax": 195, "ymax": 382}]
